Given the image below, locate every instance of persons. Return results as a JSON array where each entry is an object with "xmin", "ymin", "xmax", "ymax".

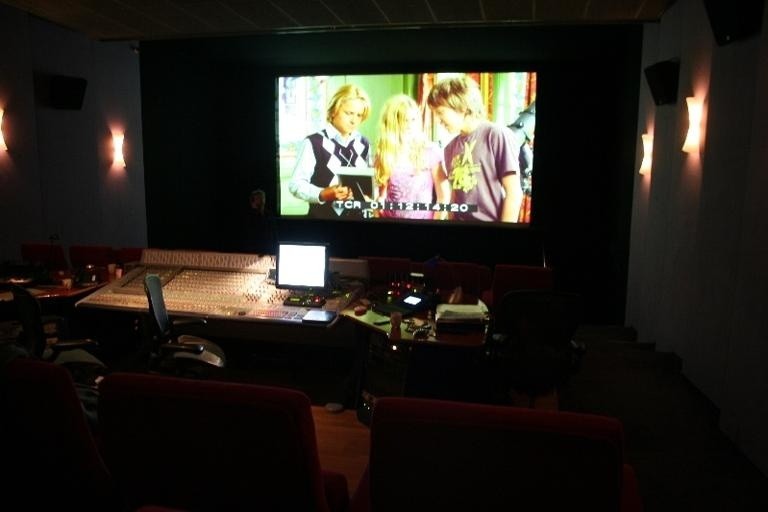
[
  {"xmin": 288, "ymin": 84, "xmax": 372, "ymax": 218},
  {"xmin": 427, "ymin": 77, "xmax": 522, "ymax": 223},
  {"xmin": 374, "ymin": 95, "xmax": 451, "ymax": 220}
]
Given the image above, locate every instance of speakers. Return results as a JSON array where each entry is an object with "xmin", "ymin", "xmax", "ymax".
[
  {"xmin": 644, "ymin": 59, "xmax": 679, "ymax": 105},
  {"xmin": 33, "ymin": 70, "xmax": 87, "ymax": 110}
]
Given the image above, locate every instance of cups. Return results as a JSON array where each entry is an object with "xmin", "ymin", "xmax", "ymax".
[{"xmin": 390, "ymin": 312, "xmax": 402, "ymax": 327}]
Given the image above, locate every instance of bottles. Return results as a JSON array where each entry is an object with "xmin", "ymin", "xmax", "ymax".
[{"xmin": 115, "ymin": 261, "xmax": 125, "ymax": 277}]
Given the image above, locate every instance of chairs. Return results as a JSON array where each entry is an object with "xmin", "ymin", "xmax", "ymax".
[{"xmin": 0, "ymin": 244, "xmax": 642, "ymax": 512}]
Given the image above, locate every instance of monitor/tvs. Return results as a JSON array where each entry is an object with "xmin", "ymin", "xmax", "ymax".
[
  {"xmin": 276, "ymin": 242, "xmax": 330, "ymax": 291},
  {"xmin": 270, "ymin": 63, "xmax": 545, "ymax": 232}
]
